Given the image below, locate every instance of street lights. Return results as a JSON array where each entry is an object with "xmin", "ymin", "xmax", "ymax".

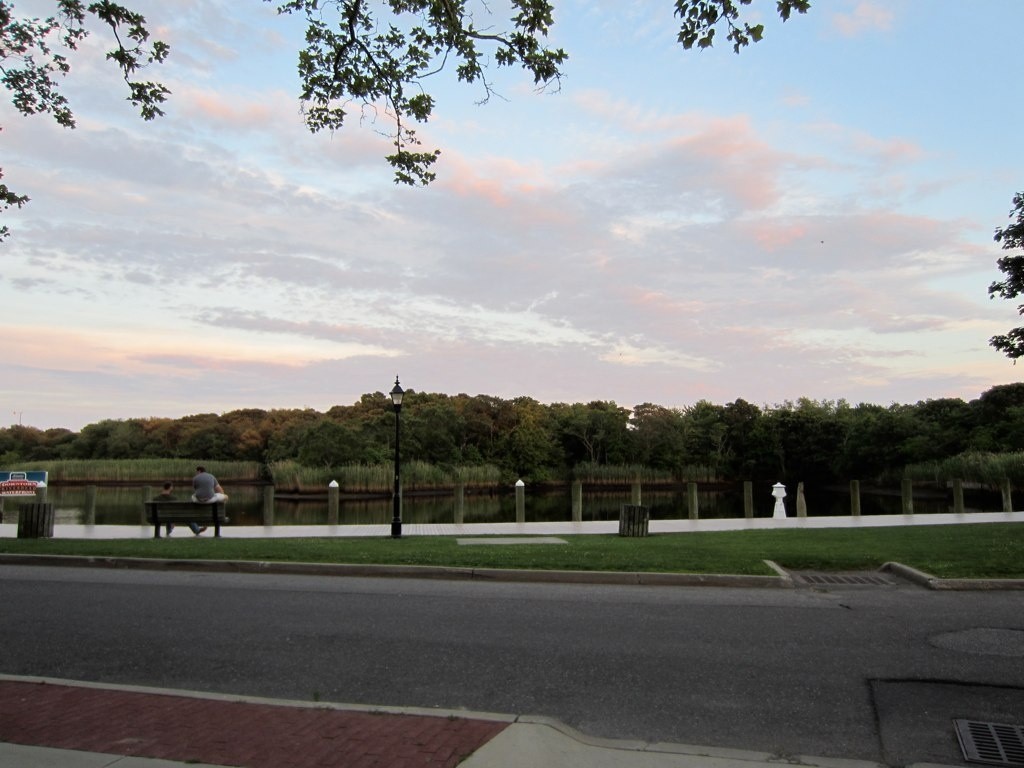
[{"xmin": 390, "ymin": 374, "xmax": 405, "ymax": 539}]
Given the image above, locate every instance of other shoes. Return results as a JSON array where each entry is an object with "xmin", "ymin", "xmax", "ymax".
[
  {"xmin": 195, "ymin": 527, "xmax": 206, "ymax": 535},
  {"xmin": 167, "ymin": 523, "xmax": 174, "ymax": 535},
  {"xmin": 225, "ymin": 517, "xmax": 229, "ymax": 520}
]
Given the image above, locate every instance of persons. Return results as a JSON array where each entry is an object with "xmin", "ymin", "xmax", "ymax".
[
  {"xmin": 191, "ymin": 466, "xmax": 229, "ymax": 505},
  {"xmin": 152, "ymin": 482, "xmax": 207, "ymax": 536}
]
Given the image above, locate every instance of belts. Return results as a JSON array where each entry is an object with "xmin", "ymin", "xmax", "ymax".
[{"xmin": 198, "ymin": 498, "xmax": 209, "ymax": 502}]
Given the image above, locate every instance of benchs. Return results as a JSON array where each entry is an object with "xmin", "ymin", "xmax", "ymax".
[{"xmin": 144, "ymin": 501, "xmax": 229, "ymax": 538}]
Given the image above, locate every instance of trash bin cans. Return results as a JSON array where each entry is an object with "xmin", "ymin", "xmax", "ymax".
[
  {"xmin": 619, "ymin": 504, "xmax": 649, "ymax": 537},
  {"xmin": 18, "ymin": 502, "xmax": 56, "ymax": 538}
]
